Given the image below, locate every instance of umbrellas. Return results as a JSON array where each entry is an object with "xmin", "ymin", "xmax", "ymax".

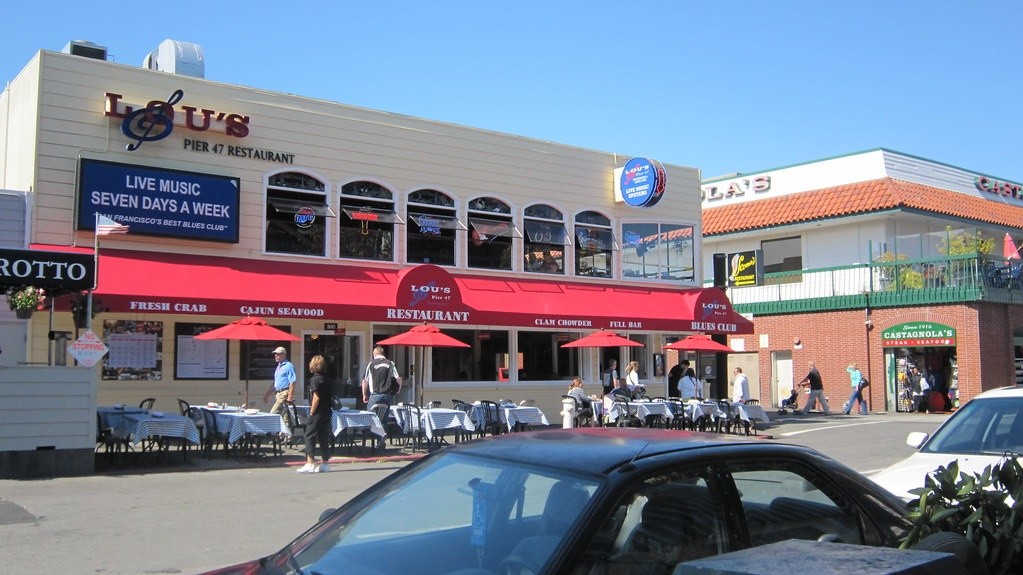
[
  {"xmin": 376, "ymin": 321, "xmax": 471, "ymax": 407},
  {"xmin": 663, "ymin": 330, "xmax": 734, "ymax": 400},
  {"xmin": 193, "ymin": 312, "xmax": 301, "ymax": 411},
  {"xmin": 1004, "ymin": 233, "xmax": 1021, "ymax": 266},
  {"xmin": 560, "ymin": 326, "xmax": 644, "ymax": 400}
]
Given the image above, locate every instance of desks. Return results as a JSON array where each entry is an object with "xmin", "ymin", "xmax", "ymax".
[
  {"xmin": 388, "ymin": 404, "xmax": 426, "ymax": 428},
  {"xmin": 719, "ymin": 403, "xmax": 769, "ymax": 436},
  {"xmin": 98, "ymin": 406, "xmax": 149, "ymax": 438},
  {"xmin": 288, "ymin": 405, "xmax": 311, "ymax": 448},
  {"xmin": 675, "ymin": 400, "xmax": 727, "ymax": 431},
  {"xmin": 331, "ymin": 409, "xmax": 387, "ymax": 454},
  {"xmin": 607, "ymin": 402, "xmax": 673, "ymax": 428},
  {"xmin": 469, "ymin": 404, "xmax": 495, "ymax": 430},
  {"xmin": 189, "ymin": 405, "xmax": 245, "ymax": 439},
  {"xmin": 408, "ymin": 408, "xmax": 476, "ymax": 448},
  {"xmin": 490, "ymin": 406, "xmax": 549, "ymax": 433},
  {"xmin": 217, "ymin": 412, "xmax": 293, "ymax": 460},
  {"xmin": 590, "ymin": 398, "xmax": 603, "ymax": 426},
  {"xmin": 124, "ymin": 413, "xmax": 201, "ymax": 465}
]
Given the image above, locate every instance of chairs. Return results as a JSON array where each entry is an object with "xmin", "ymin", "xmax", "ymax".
[
  {"xmin": 508, "ymin": 483, "xmax": 590, "ymax": 575},
  {"xmin": 95, "ymin": 397, "xmax": 536, "ymax": 472},
  {"xmin": 562, "ymin": 395, "xmax": 759, "ymax": 437},
  {"xmin": 621, "ymin": 497, "xmax": 704, "ymax": 565}
]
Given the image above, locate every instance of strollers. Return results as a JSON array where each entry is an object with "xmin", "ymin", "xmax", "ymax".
[{"xmin": 777, "ymin": 383, "xmax": 805, "ymax": 415}]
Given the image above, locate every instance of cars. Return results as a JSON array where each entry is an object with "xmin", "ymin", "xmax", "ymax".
[
  {"xmin": 852, "ymin": 385, "xmax": 1023, "ymax": 531},
  {"xmin": 194, "ymin": 431, "xmax": 922, "ymax": 575}
]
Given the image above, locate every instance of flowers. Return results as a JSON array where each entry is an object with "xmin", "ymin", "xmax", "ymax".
[{"xmin": 7, "ymin": 285, "xmax": 111, "ymax": 320}]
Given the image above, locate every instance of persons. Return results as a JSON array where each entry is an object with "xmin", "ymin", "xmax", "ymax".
[
  {"xmin": 602, "ymin": 358, "xmax": 619, "ymax": 394},
  {"xmin": 668, "ymin": 360, "xmax": 703, "ymax": 402},
  {"xmin": 782, "ymin": 390, "xmax": 796, "ymax": 408},
  {"xmin": 567, "ymin": 378, "xmax": 596, "ymax": 428},
  {"xmin": 625, "ymin": 361, "xmax": 639, "ymax": 385},
  {"xmin": 904, "ymin": 367, "xmax": 929, "ymax": 414},
  {"xmin": 799, "ymin": 361, "xmax": 830, "ymax": 415},
  {"xmin": 264, "ymin": 347, "xmax": 296, "ymax": 444},
  {"xmin": 842, "ymin": 362, "xmax": 867, "ymax": 415},
  {"xmin": 361, "ymin": 344, "xmax": 402, "ymax": 453},
  {"xmin": 731, "ymin": 367, "xmax": 750, "ymax": 415},
  {"xmin": 296, "ymin": 355, "xmax": 332, "ymax": 473}
]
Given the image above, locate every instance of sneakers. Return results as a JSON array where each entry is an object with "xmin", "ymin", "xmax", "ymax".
[
  {"xmin": 315, "ymin": 463, "xmax": 329, "ymax": 472},
  {"xmin": 296, "ymin": 463, "xmax": 316, "ymax": 473}
]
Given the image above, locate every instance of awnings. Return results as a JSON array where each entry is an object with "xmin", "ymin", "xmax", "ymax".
[{"xmin": 29, "ymin": 243, "xmax": 754, "ymax": 334}]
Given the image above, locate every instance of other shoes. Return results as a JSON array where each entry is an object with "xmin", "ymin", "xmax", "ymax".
[
  {"xmin": 842, "ymin": 411, "xmax": 849, "ymax": 415},
  {"xmin": 277, "ymin": 436, "xmax": 293, "ymax": 445},
  {"xmin": 926, "ymin": 409, "xmax": 929, "ymax": 413},
  {"xmin": 269, "ymin": 437, "xmax": 283, "ymax": 444},
  {"xmin": 799, "ymin": 409, "xmax": 808, "ymax": 416},
  {"xmin": 859, "ymin": 412, "xmax": 868, "ymax": 415},
  {"xmin": 913, "ymin": 410, "xmax": 918, "ymax": 413},
  {"xmin": 822, "ymin": 412, "xmax": 831, "ymax": 416}
]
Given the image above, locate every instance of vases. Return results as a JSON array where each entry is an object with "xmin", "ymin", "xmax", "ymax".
[{"xmin": 15, "ymin": 308, "xmax": 88, "ymax": 329}]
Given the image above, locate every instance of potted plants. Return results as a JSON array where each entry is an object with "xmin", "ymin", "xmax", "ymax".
[
  {"xmin": 875, "ymin": 252, "xmax": 908, "ymax": 291},
  {"xmin": 936, "ymin": 225, "xmax": 997, "ymax": 287}
]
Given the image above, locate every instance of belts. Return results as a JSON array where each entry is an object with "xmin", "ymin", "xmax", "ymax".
[{"xmin": 277, "ymin": 388, "xmax": 288, "ymax": 393}]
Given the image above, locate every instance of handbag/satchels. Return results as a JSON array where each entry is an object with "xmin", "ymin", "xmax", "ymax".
[
  {"xmin": 387, "ymin": 377, "xmax": 399, "ymax": 394},
  {"xmin": 329, "ymin": 392, "xmax": 342, "ymax": 410},
  {"xmin": 920, "ymin": 373, "xmax": 929, "ymax": 391},
  {"xmin": 858, "ymin": 378, "xmax": 869, "ymax": 389}
]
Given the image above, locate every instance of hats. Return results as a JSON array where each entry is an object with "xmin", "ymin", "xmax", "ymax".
[{"xmin": 272, "ymin": 346, "xmax": 286, "ymax": 354}]
